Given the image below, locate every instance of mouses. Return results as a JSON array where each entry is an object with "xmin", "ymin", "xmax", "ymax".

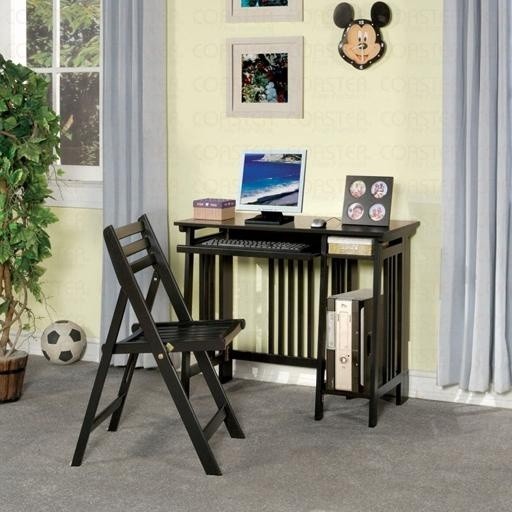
[{"xmin": 310, "ymin": 218, "xmax": 326, "ymax": 228}]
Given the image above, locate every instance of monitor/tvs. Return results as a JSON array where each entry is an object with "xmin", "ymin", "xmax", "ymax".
[{"xmin": 235, "ymin": 148, "xmax": 307, "ymax": 225}]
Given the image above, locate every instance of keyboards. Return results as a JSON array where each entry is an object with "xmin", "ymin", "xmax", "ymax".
[{"xmin": 199, "ymin": 237, "xmax": 314, "ymax": 253}]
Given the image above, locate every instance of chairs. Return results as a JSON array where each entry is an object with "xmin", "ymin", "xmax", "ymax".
[{"xmin": 70, "ymin": 214, "xmax": 246, "ymax": 475}]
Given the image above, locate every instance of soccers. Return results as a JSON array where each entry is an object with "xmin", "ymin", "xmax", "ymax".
[{"xmin": 41, "ymin": 321, "xmax": 85, "ymax": 364}]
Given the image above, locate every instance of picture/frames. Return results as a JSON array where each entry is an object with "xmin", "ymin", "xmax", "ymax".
[
  {"xmin": 340, "ymin": 173, "xmax": 394, "ymax": 227},
  {"xmin": 224, "ymin": 0, "xmax": 305, "ymax": 120}
]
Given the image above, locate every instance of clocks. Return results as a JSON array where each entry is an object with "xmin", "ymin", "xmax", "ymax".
[{"xmin": 332, "ymin": 2, "xmax": 392, "ymax": 72}]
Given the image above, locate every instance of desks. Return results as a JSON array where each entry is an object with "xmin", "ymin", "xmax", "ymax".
[{"xmin": 174, "ymin": 213, "xmax": 422, "ymax": 429}]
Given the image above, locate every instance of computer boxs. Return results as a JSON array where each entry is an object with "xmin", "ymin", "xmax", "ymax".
[{"xmin": 325, "ymin": 288, "xmax": 384, "ymax": 393}]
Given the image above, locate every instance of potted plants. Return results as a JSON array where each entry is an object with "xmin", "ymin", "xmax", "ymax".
[{"xmin": 0, "ymin": 53, "xmax": 69, "ymax": 402}]
